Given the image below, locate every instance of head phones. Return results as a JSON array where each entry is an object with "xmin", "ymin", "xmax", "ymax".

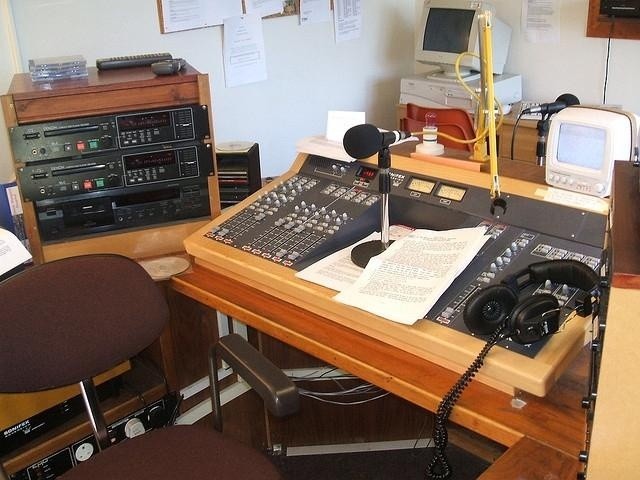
[{"xmin": 426, "ymin": 259, "xmax": 605, "ymax": 477}]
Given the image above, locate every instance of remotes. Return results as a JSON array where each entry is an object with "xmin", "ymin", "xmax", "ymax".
[
  {"xmin": 151, "ymin": 58, "xmax": 186, "ymax": 76},
  {"xmin": 96, "ymin": 53, "xmax": 171, "ymax": 71}
]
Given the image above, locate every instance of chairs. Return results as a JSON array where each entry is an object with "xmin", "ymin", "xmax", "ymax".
[{"xmin": 0, "ymin": 252, "xmax": 284, "ymax": 480}]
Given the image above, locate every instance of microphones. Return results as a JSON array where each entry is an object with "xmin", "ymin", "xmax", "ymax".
[
  {"xmin": 522, "ymin": 94, "xmax": 581, "ymax": 119},
  {"xmin": 344, "ymin": 124, "xmax": 413, "ymax": 159}
]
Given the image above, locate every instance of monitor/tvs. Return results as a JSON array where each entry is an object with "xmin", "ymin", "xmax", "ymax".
[
  {"xmin": 418, "ymin": 0, "xmax": 477, "ymax": 68},
  {"xmin": 544, "ymin": 104, "xmax": 639, "ymax": 196}
]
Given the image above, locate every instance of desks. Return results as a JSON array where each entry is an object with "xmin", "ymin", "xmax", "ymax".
[{"xmin": 172, "ymin": 134, "xmax": 638, "ymax": 479}]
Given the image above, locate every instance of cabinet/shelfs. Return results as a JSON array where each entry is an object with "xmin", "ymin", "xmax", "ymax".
[{"xmin": 0, "ymin": 61, "xmax": 221, "ymax": 390}]
